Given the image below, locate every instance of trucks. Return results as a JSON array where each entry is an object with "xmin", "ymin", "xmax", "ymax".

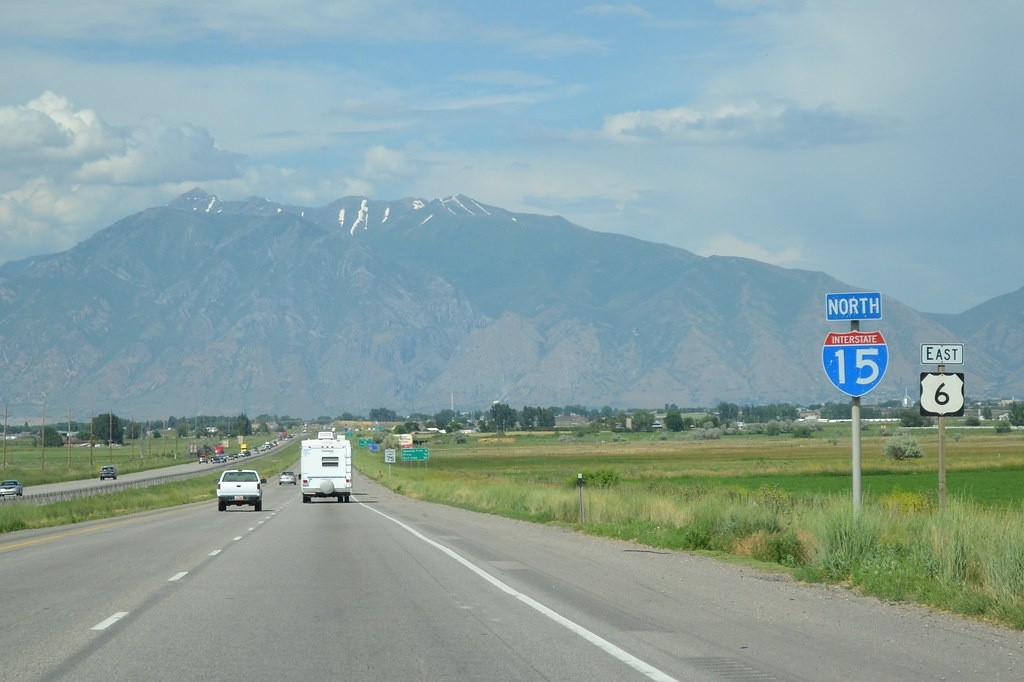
[{"xmin": 301, "ymin": 432, "xmax": 353, "ymax": 503}]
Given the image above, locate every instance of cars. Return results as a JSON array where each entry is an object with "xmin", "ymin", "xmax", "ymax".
[
  {"xmin": 214, "ymin": 470, "xmax": 267, "ymax": 511},
  {"xmin": 199, "ymin": 439, "xmax": 280, "ymax": 464},
  {"xmin": 0, "ymin": 480, "xmax": 23, "ymax": 497},
  {"xmin": 100, "ymin": 466, "xmax": 116, "ymax": 480},
  {"xmin": 278, "ymin": 471, "xmax": 298, "ymax": 486}
]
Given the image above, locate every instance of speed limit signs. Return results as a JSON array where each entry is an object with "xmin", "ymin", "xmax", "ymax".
[{"xmin": 822, "ymin": 328, "xmax": 890, "ymax": 398}]
